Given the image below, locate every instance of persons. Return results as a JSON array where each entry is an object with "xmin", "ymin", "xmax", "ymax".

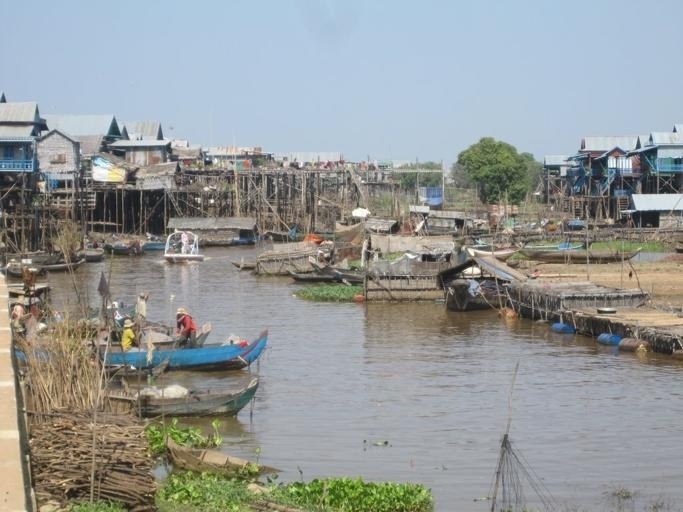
[
  {"xmin": 120, "ymin": 318, "xmax": 146, "ymax": 352},
  {"xmin": 9, "ymin": 294, "xmax": 32, "ymax": 339},
  {"xmin": 174, "ymin": 307, "xmax": 196, "ymax": 348},
  {"xmin": 106, "ymin": 301, "xmax": 122, "ymax": 320},
  {"xmin": 25, "ymin": 297, "xmax": 44, "ymax": 324},
  {"xmin": 181, "ymin": 230, "xmax": 189, "ymax": 255},
  {"xmin": 20, "ymin": 265, "xmax": 33, "ymax": 297},
  {"xmin": 135, "ymin": 290, "xmax": 150, "ymax": 322}
]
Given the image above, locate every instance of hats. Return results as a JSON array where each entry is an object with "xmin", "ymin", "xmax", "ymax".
[
  {"xmin": 123, "ymin": 319, "xmax": 136, "ymax": 328},
  {"xmin": 14, "ymin": 296, "xmax": 29, "ymax": 306},
  {"xmin": 176, "ymin": 308, "xmax": 188, "ymax": 315}
]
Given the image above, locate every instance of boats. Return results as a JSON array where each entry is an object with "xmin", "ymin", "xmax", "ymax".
[
  {"xmin": 515, "ymin": 247, "xmax": 643, "ymax": 262},
  {"xmin": 93, "ymin": 321, "xmax": 267, "ymax": 422},
  {"xmin": 284, "ymin": 267, "xmax": 363, "ymax": 281},
  {"xmin": 8, "ymin": 217, "xmax": 204, "ymax": 276}
]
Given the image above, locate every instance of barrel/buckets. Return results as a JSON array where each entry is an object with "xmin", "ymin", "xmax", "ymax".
[
  {"xmin": 597, "ymin": 332, "xmax": 620, "ymax": 343},
  {"xmin": 619, "ymin": 337, "xmax": 653, "ymax": 352},
  {"xmin": 551, "ymin": 322, "xmax": 574, "ymax": 333}
]
